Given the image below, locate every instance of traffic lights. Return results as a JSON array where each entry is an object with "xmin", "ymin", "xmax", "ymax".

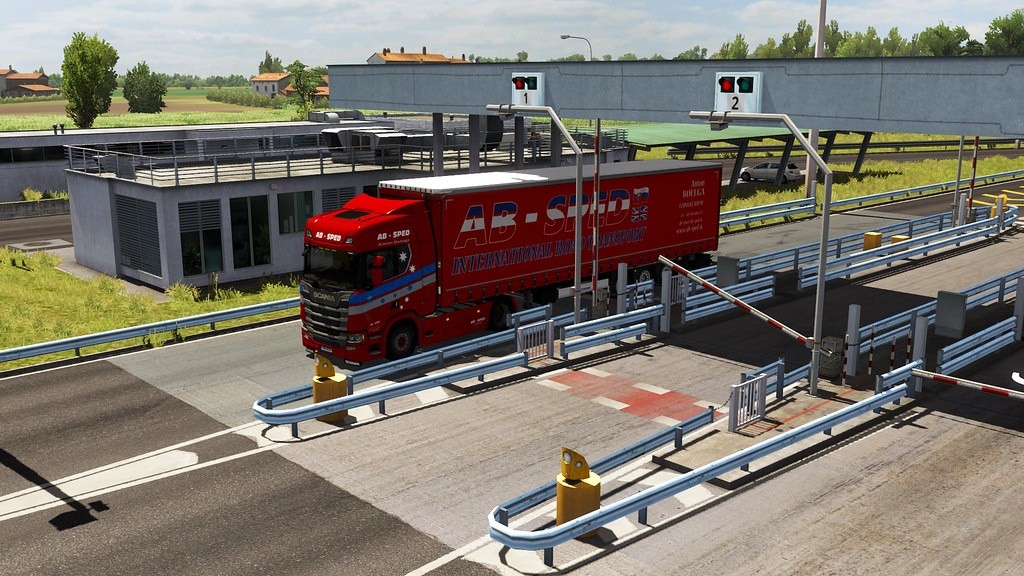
[
  {"xmin": 511, "ymin": 76, "xmax": 526, "ymax": 91},
  {"xmin": 717, "ymin": 76, "xmax": 736, "ymax": 93},
  {"xmin": 737, "ymin": 77, "xmax": 753, "ymax": 92},
  {"xmin": 521, "ymin": 76, "xmax": 537, "ymax": 91}
]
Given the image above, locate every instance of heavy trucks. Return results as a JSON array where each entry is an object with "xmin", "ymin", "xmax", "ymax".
[{"xmin": 298, "ymin": 159, "xmax": 726, "ymax": 363}]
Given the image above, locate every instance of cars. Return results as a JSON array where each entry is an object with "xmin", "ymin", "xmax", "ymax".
[{"xmin": 740, "ymin": 161, "xmax": 801, "ymax": 183}]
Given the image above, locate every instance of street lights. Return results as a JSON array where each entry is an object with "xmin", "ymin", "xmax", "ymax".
[{"xmin": 561, "ymin": 34, "xmax": 593, "ymax": 60}]
